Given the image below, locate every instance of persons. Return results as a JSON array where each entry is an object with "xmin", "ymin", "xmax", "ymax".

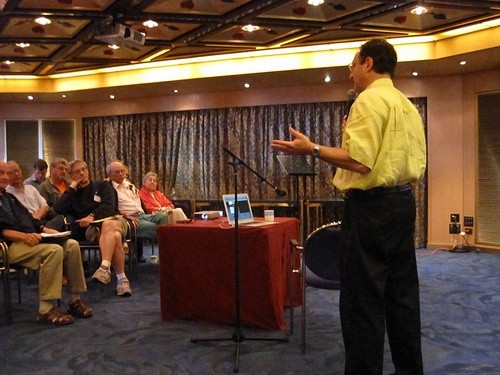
[
  {"xmin": 0, "ymin": 159, "xmax": 93, "ymax": 325},
  {"xmin": 37, "ymin": 157, "xmax": 71, "ymax": 212},
  {"xmin": 107, "ymin": 161, "xmax": 168, "ymax": 240},
  {"xmin": 270, "ymin": 38, "xmax": 429, "ymax": 375},
  {"xmin": 55, "ymin": 159, "xmax": 134, "ymax": 296},
  {"xmin": 23, "ymin": 159, "xmax": 47, "ymax": 188},
  {"xmin": 138, "ymin": 171, "xmax": 188, "ymax": 220},
  {"xmin": 5, "ymin": 160, "xmax": 69, "ymax": 285}
]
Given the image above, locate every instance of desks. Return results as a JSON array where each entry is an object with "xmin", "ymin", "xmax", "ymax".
[{"xmin": 157, "ymin": 217, "xmax": 305, "ymax": 332}]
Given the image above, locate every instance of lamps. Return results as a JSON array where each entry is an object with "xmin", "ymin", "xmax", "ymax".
[
  {"xmin": 410, "ymin": 0, "xmax": 429, "ymax": 15},
  {"xmin": 241, "ymin": 24, "xmax": 260, "ymax": 32}
]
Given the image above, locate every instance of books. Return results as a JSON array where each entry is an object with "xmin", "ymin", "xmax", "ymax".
[{"xmin": 194, "ymin": 210, "xmax": 223, "ymax": 219}]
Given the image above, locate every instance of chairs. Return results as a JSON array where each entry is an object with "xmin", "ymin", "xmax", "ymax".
[
  {"xmin": 0, "ymin": 214, "xmax": 155, "ymax": 325},
  {"xmin": 289, "ymin": 219, "xmax": 343, "ymax": 354}
]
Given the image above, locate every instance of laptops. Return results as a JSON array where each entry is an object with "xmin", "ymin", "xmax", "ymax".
[{"xmin": 221, "ymin": 191, "xmax": 279, "ymax": 228}]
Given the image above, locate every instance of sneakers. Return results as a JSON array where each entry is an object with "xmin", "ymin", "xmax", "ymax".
[
  {"xmin": 91, "ymin": 265, "xmax": 112, "ymax": 286},
  {"xmin": 116, "ymin": 277, "xmax": 133, "ymax": 296}
]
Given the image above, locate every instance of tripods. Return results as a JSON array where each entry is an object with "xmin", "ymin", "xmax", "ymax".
[{"xmin": 189, "ymin": 147, "xmax": 291, "ymax": 373}]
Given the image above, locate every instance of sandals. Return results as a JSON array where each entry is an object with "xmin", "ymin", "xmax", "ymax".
[
  {"xmin": 37, "ymin": 304, "xmax": 74, "ymax": 326},
  {"xmin": 68, "ymin": 298, "xmax": 94, "ymax": 318}
]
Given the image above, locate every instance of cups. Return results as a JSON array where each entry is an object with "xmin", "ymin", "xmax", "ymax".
[
  {"xmin": 264, "ymin": 210, "xmax": 274, "ymax": 222},
  {"xmin": 150, "ymin": 256, "xmax": 158, "ymax": 263}
]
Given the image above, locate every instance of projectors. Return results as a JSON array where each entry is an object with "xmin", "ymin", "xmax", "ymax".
[{"xmin": 94, "ymin": 24, "xmax": 145, "ymax": 48}]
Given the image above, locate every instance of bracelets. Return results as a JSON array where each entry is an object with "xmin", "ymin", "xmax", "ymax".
[{"xmin": 40, "ymin": 226, "xmax": 44, "ymax": 230}]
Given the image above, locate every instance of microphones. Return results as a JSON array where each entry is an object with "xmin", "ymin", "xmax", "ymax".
[{"xmin": 345, "ymin": 88, "xmax": 359, "ymax": 120}]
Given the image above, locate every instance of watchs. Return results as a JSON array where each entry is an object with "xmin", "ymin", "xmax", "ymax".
[
  {"xmin": 90, "ymin": 212, "xmax": 94, "ymax": 216},
  {"xmin": 311, "ymin": 144, "xmax": 321, "ymax": 157}
]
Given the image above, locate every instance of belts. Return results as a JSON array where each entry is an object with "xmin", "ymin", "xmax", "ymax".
[{"xmin": 342, "ymin": 183, "xmax": 412, "ymax": 200}]
